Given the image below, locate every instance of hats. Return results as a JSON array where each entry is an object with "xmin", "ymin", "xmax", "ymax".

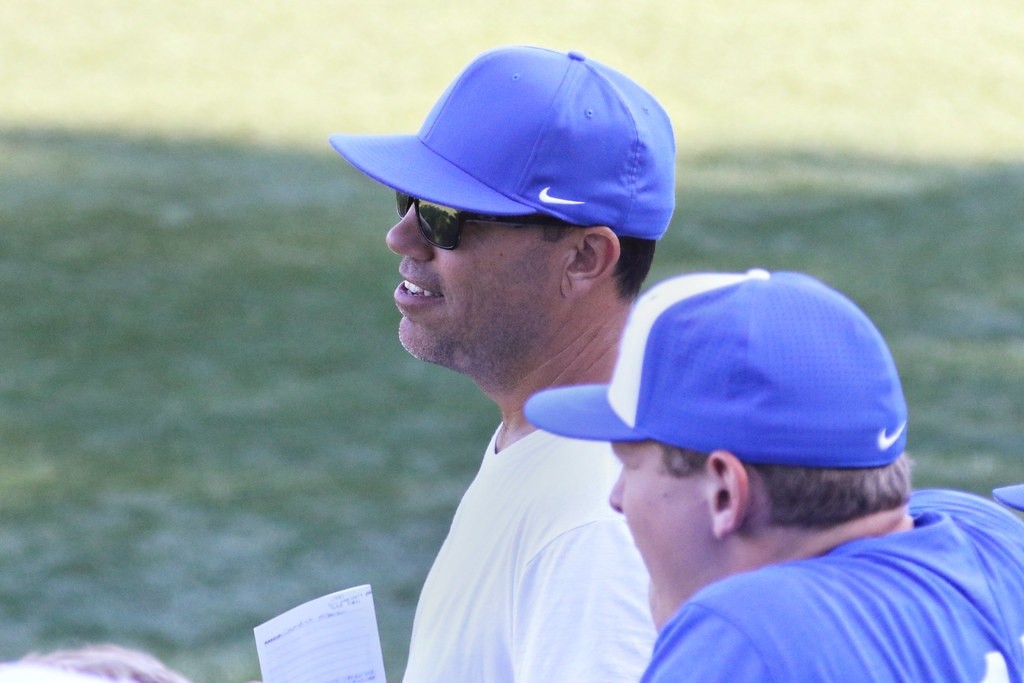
[
  {"xmin": 523, "ymin": 268, "xmax": 908, "ymax": 469},
  {"xmin": 993, "ymin": 485, "xmax": 1024, "ymax": 512},
  {"xmin": 328, "ymin": 44, "xmax": 675, "ymax": 240}
]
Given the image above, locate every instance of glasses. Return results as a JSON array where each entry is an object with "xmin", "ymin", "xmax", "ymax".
[{"xmin": 396, "ymin": 189, "xmax": 587, "ymax": 250}]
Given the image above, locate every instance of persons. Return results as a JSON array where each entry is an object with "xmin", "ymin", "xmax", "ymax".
[
  {"xmin": 524, "ymin": 270, "xmax": 1024, "ymax": 683},
  {"xmin": 328, "ymin": 44, "xmax": 675, "ymax": 683}
]
